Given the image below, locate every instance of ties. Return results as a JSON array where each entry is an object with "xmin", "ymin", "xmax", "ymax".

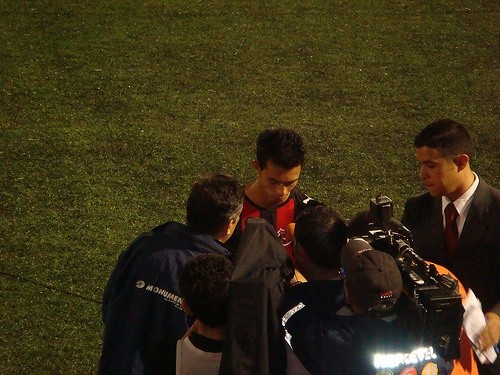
[{"xmin": 444, "ymin": 203, "xmax": 459, "ymax": 251}]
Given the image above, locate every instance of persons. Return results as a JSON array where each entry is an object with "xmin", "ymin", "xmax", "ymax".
[
  {"xmin": 400, "ymin": 118, "xmax": 500, "ymax": 375},
  {"xmin": 176, "ymin": 254, "xmax": 310, "ymax": 375},
  {"xmin": 223, "ymin": 128, "xmax": 326, "ymax": 284},
  {"xmin": 275, "ymin": 205, "xmax": 479, "ymax": 375},
  {"xmin": 99, "ymin": 174, "xmax": 244, "ymax": 375}
]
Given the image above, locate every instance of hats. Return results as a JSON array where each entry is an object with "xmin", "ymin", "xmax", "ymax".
[{"xmin": 342, "ymin": 238, "xmax": 401, "ymax": 310}]
